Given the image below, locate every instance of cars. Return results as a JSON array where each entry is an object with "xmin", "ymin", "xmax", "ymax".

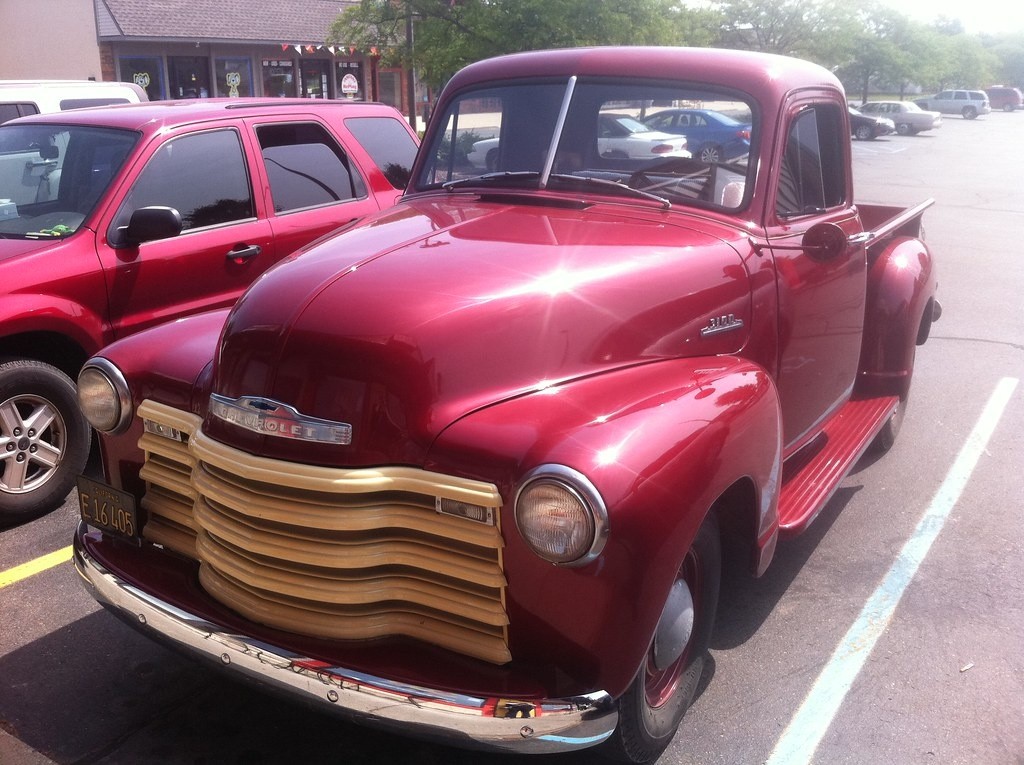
[
  {"xmin": 467, "ymin": 114, "xmax": 692, "ymax": 172},
  {"xmin": 640, "ymin": 108, "xmax": 754, "ymax": 163},
  {"xmin": 849, "ymin": 104, "xmax": 895, "ymax": 141},
  {"xmin": 913, "ymin": 89, "xmax": 989, "ymax": 121},
  {"xmin": 985, "ymin": 84, "xmax": 1024, "ymax": 112},
  {"xmin": 69, "ymin": 46, "xmax": 943, "ymax": 764},
  {"xmin": 857, "ymin": 101, "xmax": 943, "ymax": 136},
  {"xmin": 1, "ymin": 78, "xmax": 151, "ymax": 205}
]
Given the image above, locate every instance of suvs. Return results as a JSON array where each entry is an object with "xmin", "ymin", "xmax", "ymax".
[{"xmin": 1, "ymin": 96, "xmax": 422, "ymax": 516}]
